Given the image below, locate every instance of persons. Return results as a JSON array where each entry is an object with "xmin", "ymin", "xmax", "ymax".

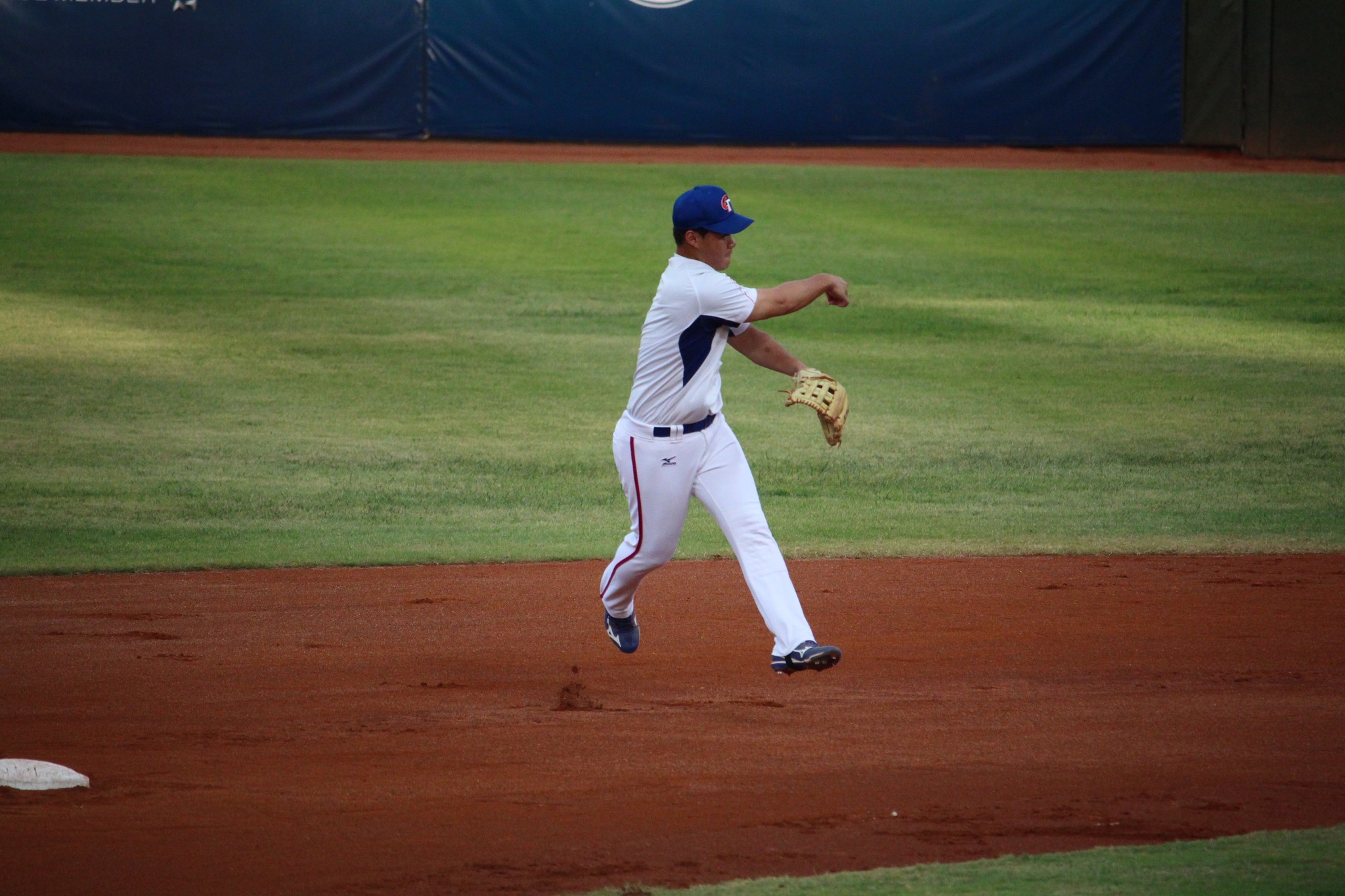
[{"xmin": 599, "ymin": 184, "xmax": 849, "ymax": 674}]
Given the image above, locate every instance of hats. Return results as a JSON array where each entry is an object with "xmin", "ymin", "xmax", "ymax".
[{"xmin": 673, "ymin": 185, "xmax": 754, "ymax": 235}]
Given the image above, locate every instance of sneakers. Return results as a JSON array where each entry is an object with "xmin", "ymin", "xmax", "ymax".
[
  {"xmin": 771, "ymin": 641, "xmax": 841, "ymax": 676},
  {"xmin": 604, "ymin": 608, "xmax": 640, "ymax": 653}
]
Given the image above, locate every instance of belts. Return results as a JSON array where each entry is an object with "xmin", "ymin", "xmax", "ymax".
[{"xmin": 653, "ymin": 413, "xmax": 716, "ymax": 437}]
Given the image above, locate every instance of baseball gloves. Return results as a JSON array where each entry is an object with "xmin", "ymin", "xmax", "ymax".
[{"xmin": 787, "ymin": 368, "xmax": 847, "ymax": 449}]
